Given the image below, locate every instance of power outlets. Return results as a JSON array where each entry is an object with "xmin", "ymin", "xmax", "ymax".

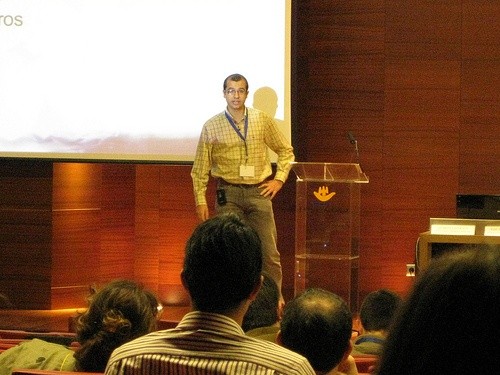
[{"xmin": 406, "ymin": 263, "xmax": 415, "ymax": 276}]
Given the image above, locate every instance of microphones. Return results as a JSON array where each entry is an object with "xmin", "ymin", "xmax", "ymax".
[{"xmin": 347, "ymin": 132, "xmax": 358, "ymax": 164}]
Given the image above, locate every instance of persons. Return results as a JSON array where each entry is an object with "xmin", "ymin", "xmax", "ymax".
[
  {"xmin": 374, "ymin": 247, "xmax": 500, "ymax": 375},
  {"xmin": 276, "ymin": 287, "xmax": 359, "ymax": 375},
  {"xmin": 192, "ymin": 74, "xmax": 294, "ymax": 296},
  {"xmin": 241, "ymin": 271, "xmax": 284, "ymax": 344},
  {"xmin": 104, "ymin": 212, "xmax": 315, "ymax": 375},
  {"xmin": 350, "ymin": 289, "xmax": 401, "ymax": 356},
  {"xmin": 0, "ymin": 278, "xmax": 163, "ymax": 375}
]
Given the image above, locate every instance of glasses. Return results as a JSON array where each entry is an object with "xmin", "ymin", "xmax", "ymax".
[{"xmin": 226, "ymin": 89, "xmax": 247, "ymax": 95}]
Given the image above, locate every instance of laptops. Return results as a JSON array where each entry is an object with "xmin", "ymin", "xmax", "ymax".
[{"xmin": 456, "ymin": 194, "xmax": 500, "ymax": 220}]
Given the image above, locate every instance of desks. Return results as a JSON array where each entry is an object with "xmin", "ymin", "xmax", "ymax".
[{"xmin": 420, "ymin": 231, "xmax": 500, "ymax": 267}]
